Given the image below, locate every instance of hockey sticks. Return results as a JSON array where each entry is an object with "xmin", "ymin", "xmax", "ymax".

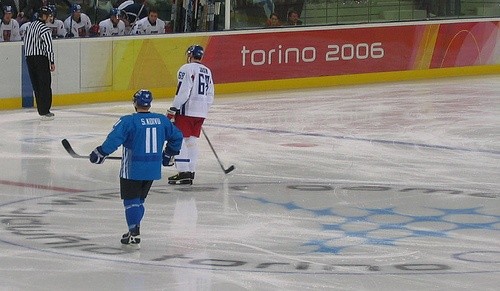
[
  {"xmin": 62, "ymin": 138, "xmax": 191, "ymax": 163},
  {"xmin": 201, "ymin": 128, "xmax": 235, "ymax": 174}
]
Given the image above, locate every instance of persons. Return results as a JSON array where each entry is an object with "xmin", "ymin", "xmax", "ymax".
[
  {"xmin": 89, "ymin": 90, "xmax": 183, "ymax": 244},
  {"xmin": 0, "ymin": 4, "xmax": 166, "ymax": 42},
  {"xmin": 284, "ymin": 10, "xmax": 303, "ymax": 26},
  {"xmin": 24, "ymin": 7, "xmax": 55, "ymax": 117},
  {"xmin": 266, "ymin": 12, "xmax": 281, "ymax": 26},
  {"xmin": 167, "ymin": 45, "xmax": 215, "ymax": 180}
]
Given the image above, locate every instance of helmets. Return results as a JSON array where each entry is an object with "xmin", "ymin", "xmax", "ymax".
[
  {"xmin": 2, "ymin": 6, "xmax": 14, "ymax": 15},
  {"xmin": 47, "ymin": 3, "xmax": 57, "ymax": 15},
  {"xmin": 71, "ymin": 4, "xmax": 82, "ymax": 12},
  {"xmin": 132, "ymin": 88, "xmax": 153, "ymax": 107},
  {"xmin": 110, "ymin": 8, "xmax": 121, "ymax": 16},
  {"xmin": 38, "ymin": 6, "xmax": 53, "ymax": 15},
  {"xmin": 185, "ymin": 45, "xmax": 204, "ymax": 58}
]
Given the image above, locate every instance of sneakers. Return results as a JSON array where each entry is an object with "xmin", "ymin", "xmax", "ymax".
[
  {"xmin": 120, "ymin": 230, "xmax": 141, "ymax": 248},
  {"xmin": 168, "ymin": 171, "xmax": 196, "ymax": 185}
]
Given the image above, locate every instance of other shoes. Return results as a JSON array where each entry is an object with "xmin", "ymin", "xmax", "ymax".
[{"xmin": 40, "ymin": 113, "xmax": 56, "ymax": 120}]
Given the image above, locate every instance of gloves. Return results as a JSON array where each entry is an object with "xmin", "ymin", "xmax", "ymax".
[
  {"xmin": 161, "ymin": 145, "xmax": 180, "ymax": 167},
  {"xmin": 89, "ymin": 146, "xmax": 109, "ymax": 166},
  {"xmin": 165, "ymin": 109, "xmax": 177, "ymax": 125}
]
